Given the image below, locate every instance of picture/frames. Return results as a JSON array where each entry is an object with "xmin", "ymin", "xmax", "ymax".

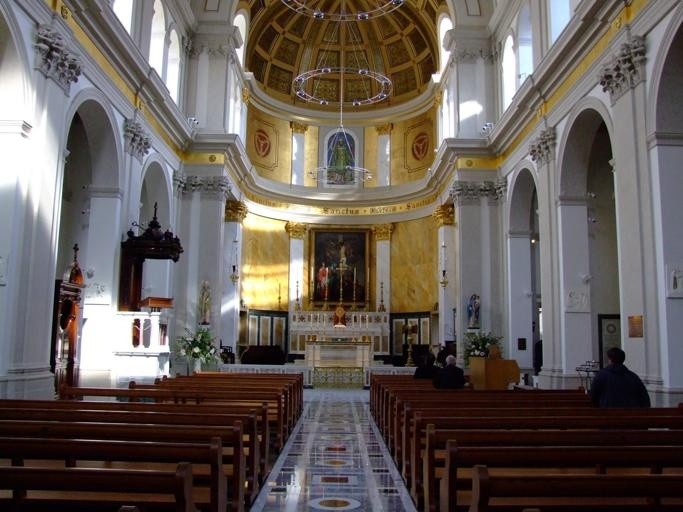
[{"xmin": 598, "ymin": 313, "xmax": 621, "ymax": 371}]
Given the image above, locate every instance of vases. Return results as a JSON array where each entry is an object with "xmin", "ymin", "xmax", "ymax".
[{"xmin": 193, "ymin": 358, "xmax": 201, "ymax": 372}]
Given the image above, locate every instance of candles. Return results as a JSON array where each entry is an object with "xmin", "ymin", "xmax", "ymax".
[
  {"xmin": 325, "ymin": 267, "xmax": 328, "ymax": 288},
  {"xmin": 366, "ymin": 280, "xmax": 370, "ymax": 298},
  {"xmin": 296, "ymin": 280, "xmax": 299, "ymax": 298},
  {"xmin": 380, "ymin": 282, "xmax": 384, "ymax": 301},
  {"xmin": 353, "ymin": 267, "xmax": 357, "ymax": 288},
  {"xmin": 339, "ymin": 263, "xmax": 343, "ymax": 289},
  {"xmin": 310, "ymin": 281, "xmax": 314, "ymax": 296},
  {"xmin": 278, "ymin": 283, "xmax": 281, "ymax": 297}
]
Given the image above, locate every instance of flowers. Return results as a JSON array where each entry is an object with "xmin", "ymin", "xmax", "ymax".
[
  {"xmin": 175, "ymin": 327, "xmax": 220, "ymax": 364},
  {"xmin": 460, "ymin": 331, "xmax": 504, "ymax": 360}
]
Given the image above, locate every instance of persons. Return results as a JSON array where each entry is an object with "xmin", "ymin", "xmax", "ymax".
[
  {"xmin": 588, "ymin": 347, "xmax": 651, "ymax": 430},
  {"xmin": 432, "ymin": 354, "xmax": 466, "ymax": 389},
  {"xmin": 415, "ymin": 354, "xmax": 440, "ymax": 378}
]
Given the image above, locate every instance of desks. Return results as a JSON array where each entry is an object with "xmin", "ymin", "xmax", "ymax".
[{"xmin": 469, "ymin": 357, "xmax": 520, "ymax": 390}]
